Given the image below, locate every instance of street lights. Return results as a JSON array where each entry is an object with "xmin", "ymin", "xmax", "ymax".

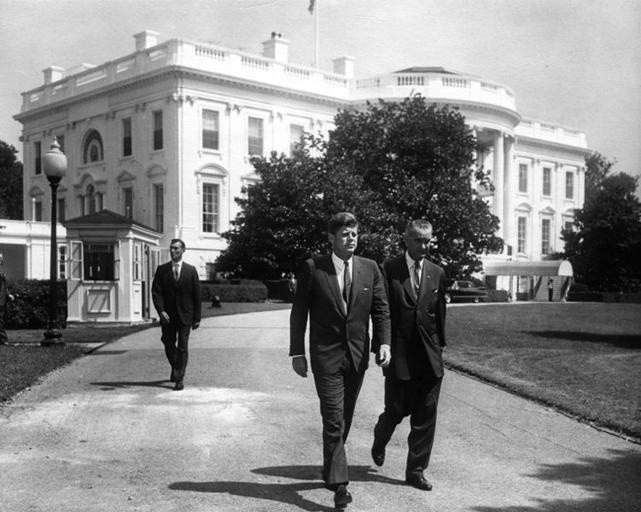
[{"xmin": 38, "ymin": 134, "xmax": 70, "ymax": 349}]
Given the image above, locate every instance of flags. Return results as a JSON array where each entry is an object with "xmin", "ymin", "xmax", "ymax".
[{"xmin": 308, "ymin": 0, "xmax": 316, "ymax": 17}]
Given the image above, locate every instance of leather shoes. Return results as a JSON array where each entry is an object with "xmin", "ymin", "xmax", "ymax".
[
  {"xmin": 332, "ymin": 488, "xmax": 354, "ymax": 510},
  {"xmin": 169, "ymin": 368, "xmax": 184, "ymax": 391},
  {"xmin": 407, "ymin": 469, "xmax": 433, "ymax": 492},
  {"xmin": 372, "ymin": 434, "xmax": 387, "ymax": 468}
]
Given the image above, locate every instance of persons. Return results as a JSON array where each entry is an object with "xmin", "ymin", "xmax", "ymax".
[
  {"xmin": 150, "ymin": 237, "xmax": 201, "ymax": 393},
  {"xmin": 285, "ymin": 209, "xmax": 394, "ymax": 512},
  {"xmin": 546, "ymin": 279, "xmax": 556, "ymax": 303},
  {"xmin": 287, "ymin": 273, "xmax": 297, "ymax": 298},
  {"xmin": 370, "ymin": 218, "xmax": 451, "ymax": 492},
  {"xmin": 0, "ymin": 252, "xmax": 16, "ymax": 347}
]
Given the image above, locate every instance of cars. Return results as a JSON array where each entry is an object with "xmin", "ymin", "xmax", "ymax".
[{"xmin": 442, "ymin": 278, "xmax": 490, "ymax": 304}]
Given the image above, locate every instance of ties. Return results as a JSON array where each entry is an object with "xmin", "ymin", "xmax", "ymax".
[
  {"xmin": 413, "ymin": 263, "xmax": 423, "ymax": 292},
  {"xmin": 342, "ymin": 262, "xmax": 355, "ymax": 303},
  {"xmin": 174, "ymin": 266, "xmax": 178, "ymax": 277}
]
